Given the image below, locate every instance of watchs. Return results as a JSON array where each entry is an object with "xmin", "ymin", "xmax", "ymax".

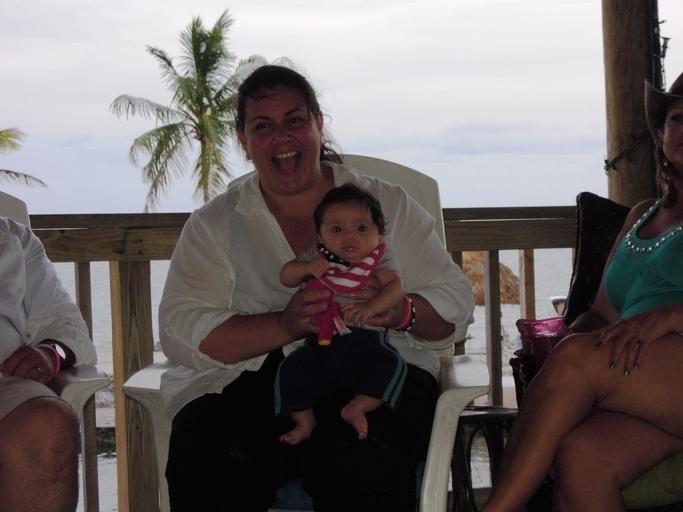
[{"xmin": 49, "ymin": 342, "xmax": 66, "ymax": 361}]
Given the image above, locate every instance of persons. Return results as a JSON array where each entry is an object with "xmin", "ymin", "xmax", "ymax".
[
  {"xmin": 159, "ymin": 65, "xmax": 475, "ymax": 512},
  {"xmin": 274, "ymin": 182, "xmax": 409, "ymax": 445},
  {"xmin": 477, "ymin": 69, "xmax": 683, "ymax": 512},
  {"xmin": 0, "ymin": 214, "xmax": 94, "ymax": 512}
]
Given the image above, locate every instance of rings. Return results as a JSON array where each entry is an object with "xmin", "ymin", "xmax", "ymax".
[{"xmin": 33, "ymin": 366, "xmax": 44, "ymax": 374}]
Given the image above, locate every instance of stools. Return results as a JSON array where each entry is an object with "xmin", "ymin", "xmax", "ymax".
[{"xmin": 447, "ymin": 404, "xmax": 518, "ymax": 512}]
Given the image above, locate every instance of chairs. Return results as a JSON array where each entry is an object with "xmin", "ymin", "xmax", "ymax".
[
  {"xmin": 509, "ymin": 190, "xmax": 682, "ymax": 512},
  {"xmin": 122, "ymin": 153, "xmax": 492, "ymax": 512}
]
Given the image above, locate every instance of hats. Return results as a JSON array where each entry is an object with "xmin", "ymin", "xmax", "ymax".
[{"xmin": 644, "ymin": 72, "xmax": 682, "ymax": 140}]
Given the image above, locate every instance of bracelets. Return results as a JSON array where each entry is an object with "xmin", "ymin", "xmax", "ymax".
[
  {"xmin": 34, "ymin": 343, "xmax": 62, "ymax": 379},
  {"xmin": 391, "ymin": 294, "xmax": 417, "ymax": 333}
]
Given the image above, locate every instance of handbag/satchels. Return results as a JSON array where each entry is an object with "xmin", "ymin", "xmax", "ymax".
[{"xmin": 514, "ymin": 318, "xmax": 568, "ymax": 366}]
